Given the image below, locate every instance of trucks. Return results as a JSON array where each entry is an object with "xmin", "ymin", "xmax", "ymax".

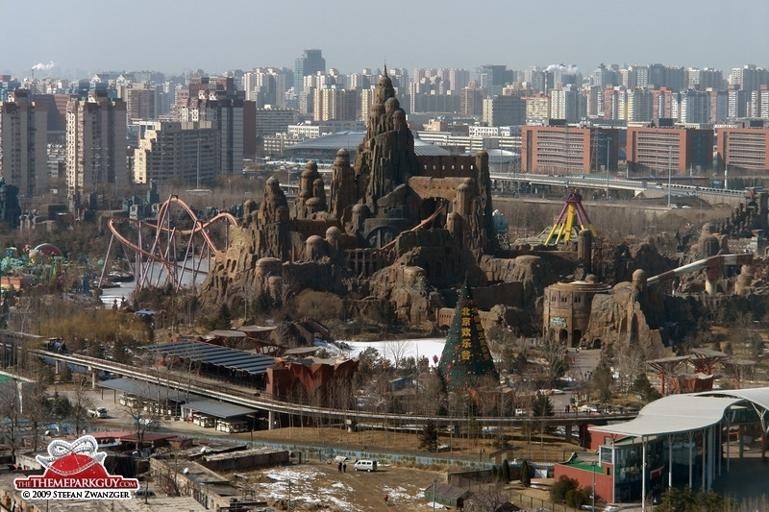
[{"xmin": 88, "ymin": 407, "xmax": 107, "ymax": 419}]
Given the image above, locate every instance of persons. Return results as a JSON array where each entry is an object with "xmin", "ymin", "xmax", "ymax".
[
  {"xmin": 338, "ymin": 463, "xmax": 340, "ymax": 472},
  {"xmin": 339, "ymin": 461, "xmax": 342, "ymax": 470},
  {"xmin": 343, "ymin": 462, "xmax": 347, "ymax": 473}
]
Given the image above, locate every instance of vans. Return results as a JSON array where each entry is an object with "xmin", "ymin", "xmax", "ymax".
[{"xmin": 353, "ymin": 460, "xmax": 377, "ymax": 473}]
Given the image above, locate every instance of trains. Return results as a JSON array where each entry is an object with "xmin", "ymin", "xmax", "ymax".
[{"xmin": 0, "ymin": 329, "xmax": 66, "ymax": 355}]
